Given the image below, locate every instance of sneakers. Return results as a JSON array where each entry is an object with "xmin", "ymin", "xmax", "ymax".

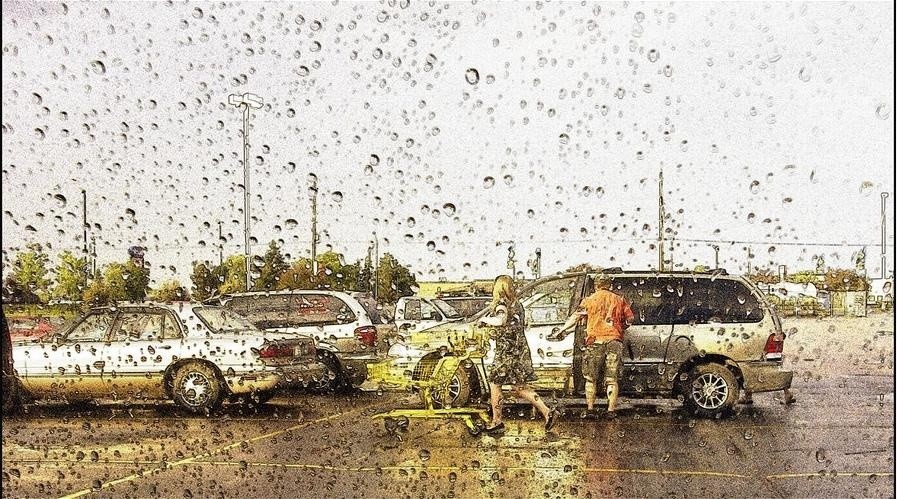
[
  {"xmin": 587, "ymin": 409, "xmax": 598, "ymax": 420},
  {"xmin": 543, "ymin": 409, "xmax": 560, "ymax": 430},
  {"xmin": 486, "ymin": 421, "xmax": 506, "ymax": 434},
  {"xmin": 605, "ymin": 410, "xmax": 618, "ymax": 419}
]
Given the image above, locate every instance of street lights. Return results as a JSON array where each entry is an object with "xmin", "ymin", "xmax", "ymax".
[{"xmin": 225, "ymin": 91, "xmax": 264, "ymax": 292}]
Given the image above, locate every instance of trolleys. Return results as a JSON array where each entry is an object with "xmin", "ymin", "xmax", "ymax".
[{"xmin": 372, "ymin": 325, "xmax": 492, "ymax": 436}]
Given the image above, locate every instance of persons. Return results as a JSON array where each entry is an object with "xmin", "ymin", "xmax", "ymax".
[
  {"xmin": 548, "ymin": 273, "xmax": 635, "ymax": 420},
  {"xmin": 737, "ymin": 387, "xmax": 797, "ymax": 406},
  {"xmin": 476, "ymin": 272, "xmax": 561, "ymax": 434}
]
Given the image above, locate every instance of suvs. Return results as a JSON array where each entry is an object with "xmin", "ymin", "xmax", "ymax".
[{"xmin": 0, "ymin": 269, "xmax": 793, "ymax": 420}]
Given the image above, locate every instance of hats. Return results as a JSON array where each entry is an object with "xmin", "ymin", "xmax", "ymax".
[{"xmin": 594, "ymin": 272, "xmax": 612, "ymax": 285}]
